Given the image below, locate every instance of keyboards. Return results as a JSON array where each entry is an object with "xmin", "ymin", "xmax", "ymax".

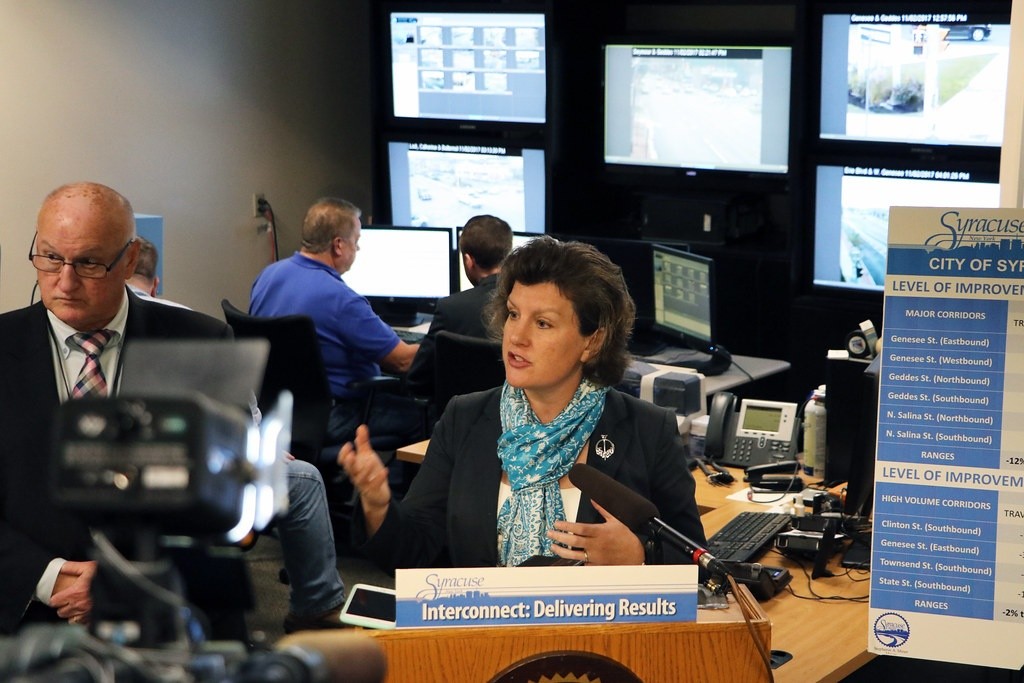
[{"xmin": 701, "ymin": 512, "xmax": 797, "ymax": 563}]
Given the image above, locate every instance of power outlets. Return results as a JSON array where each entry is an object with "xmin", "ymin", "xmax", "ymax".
[{"xmin": 254, "ymin": 191, "xmax": 270, "ymax": 216}]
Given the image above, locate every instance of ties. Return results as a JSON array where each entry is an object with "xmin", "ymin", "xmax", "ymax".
[{"xmin": 63, "ymin": 330, "xmax": 116, "ymax": 401}]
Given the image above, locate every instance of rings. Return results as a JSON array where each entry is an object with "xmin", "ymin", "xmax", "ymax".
[{"xmin": 584, "ymin": 550, "xmax": 588, "ymax": 563}]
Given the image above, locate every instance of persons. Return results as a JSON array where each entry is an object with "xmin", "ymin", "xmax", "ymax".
[
  {"xmin": 124, "ymin": 234, "xmax": 358, "ymax": 637},
  {"xmin": 406, "ymin": 214, "xmax": 513, "ymax": 439},
  {"xmin": 337, "ymin": 234, "xmax": 709, "ymax": 583},
  {"xmin": 248, "ymin": 195, "xmax": 421, "ymax": 440},
  {"xmin": 1, "ymin": 180, "xmax": 260, "ymax": 639}
]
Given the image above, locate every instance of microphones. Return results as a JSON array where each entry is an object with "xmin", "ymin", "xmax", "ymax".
[{"xmin": 567, "ymin": 462, "xmax": 733, "ymax": 578}]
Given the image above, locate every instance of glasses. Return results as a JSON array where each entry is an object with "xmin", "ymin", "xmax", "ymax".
[{"xmin": 28, "ymin": 231, "xmax": 134, "ymax": 279}]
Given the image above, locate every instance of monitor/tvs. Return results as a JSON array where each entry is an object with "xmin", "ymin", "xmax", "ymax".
[
  {"xmin": 456, "ymin": 226, "xmax": 731, "ymax": 377},
  {"xmin": 390, "ymin": 11, "xmax": 552, "ymax": 134},
  {"xmin": 820, "ymin": 11, "xmax": 1012, "ymax": 149},
  {"xmin": 385, "ymin": 138, "xmax": 551, "ymax": 237},
  {"xmin": 811, "ymin": 165, "xmax": 999, "ymax": 292},
  {"xmin": 839, "ymin": 355, "xmax": 882, "ymax": 569},
  {"xmin": 340, "ymin": 226, "xmax": 453, "ymax": 327},
  {"xmin": 602, "ymin": 43, "xmax": 792, "ymax": 179}
]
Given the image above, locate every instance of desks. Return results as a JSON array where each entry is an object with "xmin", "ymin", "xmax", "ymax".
[{"xmin": 398, "ymin": 424, "xmax": 895, "ymax": 683}]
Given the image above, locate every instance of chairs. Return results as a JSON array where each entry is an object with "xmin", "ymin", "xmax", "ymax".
[
  {"xmin": 220, "ymin": 301, "xmax": 429, "ymax": 529},
  {"xmin": 424, "ymin": 331, "xmax": 508, "ymax": 433}
]
